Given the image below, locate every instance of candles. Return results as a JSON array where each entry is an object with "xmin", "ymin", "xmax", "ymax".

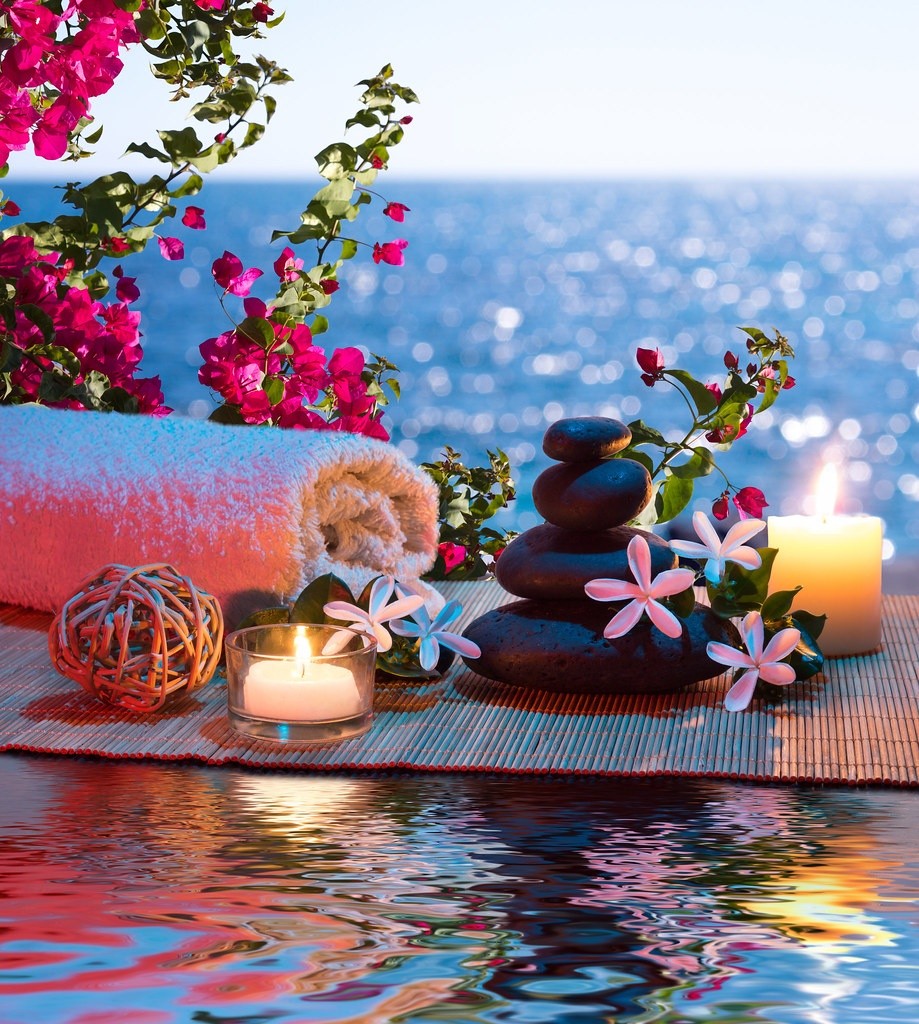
[
  {"xmin": 226, "ymin": 612, "xmax": 379, "ymax": 730},
  {"xmin": 764, "ymin": 459, "xmax": 885, "ymax": 658}
]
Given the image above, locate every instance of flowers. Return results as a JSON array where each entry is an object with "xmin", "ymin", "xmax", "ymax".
[
  {"xmin": 588, "ymin": 324, "xmax": 826, "ymax": 714},
  {"xmin": 255, "ymin": 568, "xmax": 482, "ymax": 678}
]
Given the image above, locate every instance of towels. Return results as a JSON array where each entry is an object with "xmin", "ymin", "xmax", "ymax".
[{"xmin": 0, "ymin": 402, "xmax": 440, "ymax": 617}]
